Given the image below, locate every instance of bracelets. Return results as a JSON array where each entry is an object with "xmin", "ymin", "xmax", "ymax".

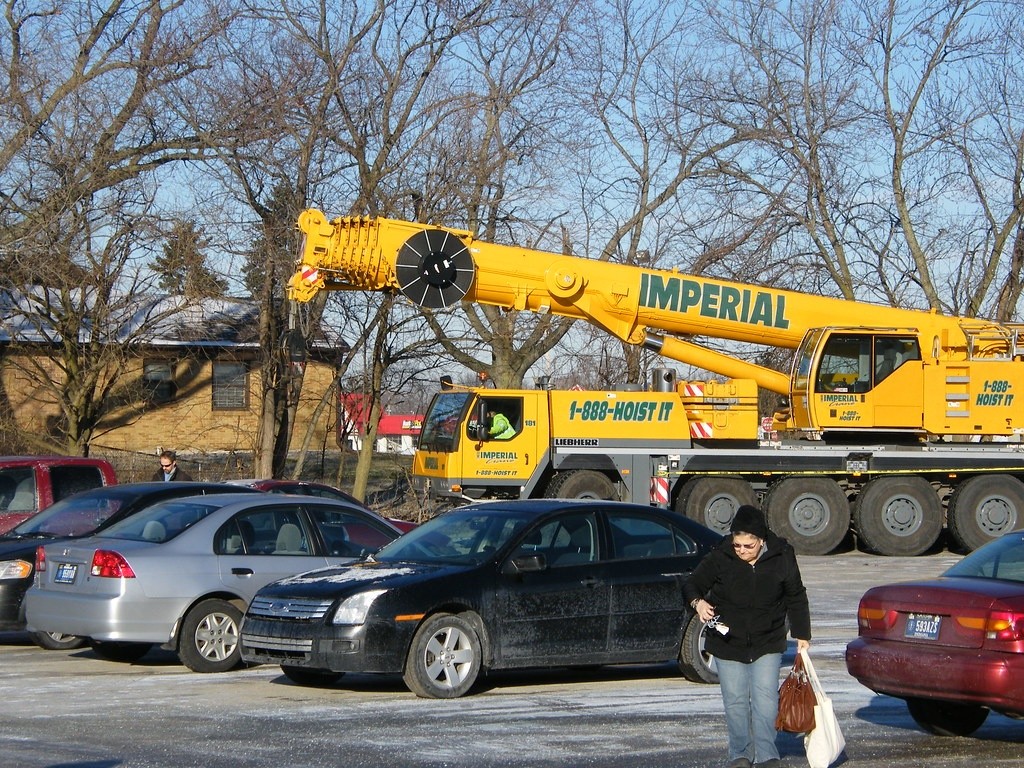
[{"xmin": 691, "ymin": 598, "xmax": 704, "ymax": 611}]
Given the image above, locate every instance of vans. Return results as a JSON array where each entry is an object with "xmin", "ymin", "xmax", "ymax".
[{"xmin": 2, "ymin": 457, "xmax": 120, "ymax": 547}]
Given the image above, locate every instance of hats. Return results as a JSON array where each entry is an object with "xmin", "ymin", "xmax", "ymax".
[{"xmin": 730, "ymin": 506, "xmax": 767, "ymax": 539}]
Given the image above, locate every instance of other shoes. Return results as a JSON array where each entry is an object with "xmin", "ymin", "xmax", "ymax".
[
  {"xmin": 731, "ymin": 758, "xmax": 750, "ymax": 768},
  {"xmin": 756, "ymin": 759, "xmax": 788, "ymax": 768}
]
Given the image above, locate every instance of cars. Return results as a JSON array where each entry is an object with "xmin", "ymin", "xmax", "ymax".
[
  {"xmin": 238, "ymin": 500, "xmax": 731, "ymax": 699},
  {"xmin": 846, "ymin": 530, "xmax": 1024, "ymax": 736},
  {"xmin": 228, "ymin": 474, "xmax": 357, "ymax": 499},
  {"xmin": 0, "ymin": 481, "xmax": 250, "ymax": 650},
  {"xmin": 24, "ymin": 493, "xmax": 404, "ymax": 670}
]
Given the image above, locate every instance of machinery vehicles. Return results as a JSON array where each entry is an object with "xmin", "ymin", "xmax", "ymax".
[{"xmin": 286, "ymin": 206, "xmax": 1024, "ymax": 553}]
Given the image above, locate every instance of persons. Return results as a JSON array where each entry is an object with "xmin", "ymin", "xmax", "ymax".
[
  {"xmin": 682, "ymin": 505, "xmax": 811, "ymax": 768},
  {"xmin": 487, "ymin": 408, "xmax": 516, "ymax": 440},
  {"xmin": 151, "ymin": 451, "xmax": 193, "ymax": 481}
]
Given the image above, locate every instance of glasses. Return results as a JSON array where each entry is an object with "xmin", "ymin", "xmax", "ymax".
[
  {"xmin": 159, "ymin": 462, "xmax": 173, "ymax": 467},
  {"xmin": 730, "ymin": 538, "xmax": 759, "ymax": 549}
]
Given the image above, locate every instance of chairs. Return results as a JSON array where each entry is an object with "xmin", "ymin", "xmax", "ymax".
[
  {"xmin": 141, "ymin": 521, "xmax": 166, "ymax": 541},
  {"xmin": 7, "ymin": 476, "xmax": 34, "ymax": 510},
  {"xmin": 272, "ymin": 524, "xmax": 307, "ymax": 554},
  {"xmin": 223, "ymin": 520, "xmax": 258, "ymax": 553},
  {"xmin": 509, "ymin": 521, "xmax": 547, "ymax": 569},
  {"xmin": 551, "ymin": 525, "xmax": 590, "ymax": 565}
]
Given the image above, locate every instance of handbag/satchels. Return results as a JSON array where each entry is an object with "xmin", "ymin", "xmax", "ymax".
[
  {"xmin": 800, "ymin": 648, "xmax": 846, "ymax": 768},
  {"xmin": 775, "ymin": 652, "xmax": 818, "ymax": 732}
]
